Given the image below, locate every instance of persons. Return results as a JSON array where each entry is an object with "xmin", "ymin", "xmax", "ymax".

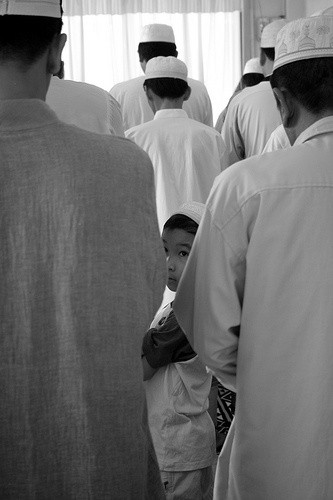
[
  {"xmin": 215, "ymin": 57, "xmax": 266, "ymax": 133},
  {"xmin": 45, "ymin": 61, "xmax": 124, "ymax": 137},
  {"xmin": 124, "ymin": 56, "xmax": 226, "ymax": 452},
  {"xmin": 221, "ymin": 18, "xmax": 293, "ymax": 168},
  {"xmin": 141, "ymin": 211, "xmax": 217, "ymax": 499},
  {"xmin": 108, "ymin": 23, "xmax": 213, "ymax": 134},
  {"xmin": 0, "ymin": 0, "xmax": 169, "ymax": 500},
  {"xmin": 172, "ymin": 14, "xmax": 333, "ymax": 500}
]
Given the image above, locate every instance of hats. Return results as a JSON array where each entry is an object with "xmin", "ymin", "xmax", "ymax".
[
  {"xmin": 0, "ymin": 0, "xmax": 63, "ymax": 19},
  {"xmin": 261, "ymin": 19, "xmax": 291, "ymax": 48},
  {"xmin": 139, "ymin": 24, "xmax": 175, "ymax": 43},
  {"xmin": 272, "ymin": 15, "xmax": 333, "ymax": 72},
  {"xmin": 243, "ymin": 57, "xmax": 264, "ymax": 75},
  {"xmin": 175, "ymin": 201, "xmax": 206, "ymax": 226},
  {"xmin": 144, "ymin": 56, "xmax": 188, "ymax": 84}
]
